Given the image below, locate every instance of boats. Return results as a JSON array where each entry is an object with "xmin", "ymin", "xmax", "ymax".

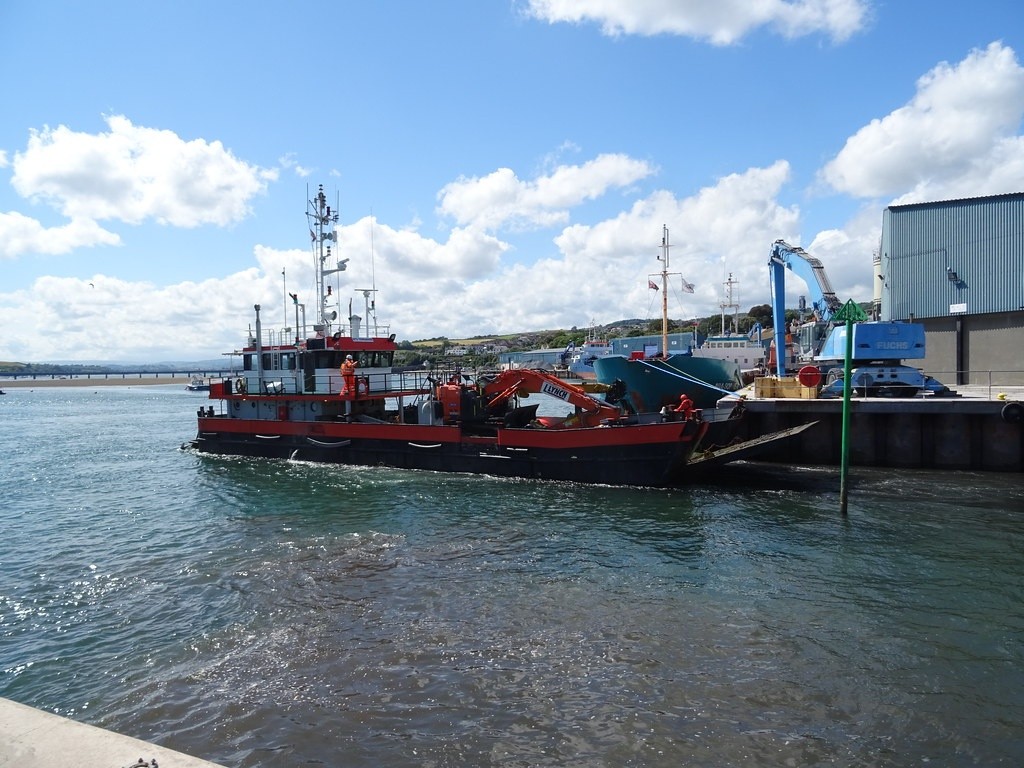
[
  {"xmin": 197, "ymin": 182, "xmax": 821, "ymax": 487},
  {"xmin": 188, "ymin": 375, "xmax": 209, "ymax": 391},
  {"xmin": 594, "ymin": 222, "xmax": 745, "ymax": 412},
  {"xmin": 568, "ymin": 325, "xmax": 614, "ymax": 373},
  {"xmin": 690, "ymin": 273, "xmax": 765, "ymax": 385}
]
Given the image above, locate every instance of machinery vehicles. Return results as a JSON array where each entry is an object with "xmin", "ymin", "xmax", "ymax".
[
  {"xmin": 555, "ymin": 341, "xmax": 576, "ymax": 369},
  {"xmin": 768, "ymin": 238, "xmax": 962, "ymax": 399}
]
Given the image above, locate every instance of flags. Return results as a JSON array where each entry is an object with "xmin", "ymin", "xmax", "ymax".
[
  {"xmin": 649, "ymin": 280, "xmax": 659, "ymax": 290},
  {"xmin": 682, "ymin": 279, "xmax": 695, "ymax": 293}
]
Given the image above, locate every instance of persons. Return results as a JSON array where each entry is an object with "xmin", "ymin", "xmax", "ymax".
[
  {"xmin": 340, "ymin": 355, "xmax": 358, "ymax": 396},
  {"xmin": 674, "ymin": 394, "xmax": 694, "ymax": 421}
]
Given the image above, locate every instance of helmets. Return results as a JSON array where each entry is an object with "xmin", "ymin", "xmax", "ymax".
[
  {"xmin": 681, "ymin": 394, "xmax": 686, "ymax": 399},
  {"xmin": 736, "ymin": 398, "xmax": 743, "ymax": 402},
  {"xmin": 346, "ymin": 355, "xmax": 352, "ymax": 359}
]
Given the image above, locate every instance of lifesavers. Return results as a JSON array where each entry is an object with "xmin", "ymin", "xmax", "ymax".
[
  {"xmin": 235, "ymin": 378, "xmax": 246, "ymax": 393},
  {"xmin": 421, "ymin": 400, "xmax": 442, "ymax": 426},
  {"xmin": 355, "ymin": 380, "xmax": 369, "ymax": 397},
  {"xmin": 1000, "ymin": 402, "xmax": 1024, "ymax": 426}
]
